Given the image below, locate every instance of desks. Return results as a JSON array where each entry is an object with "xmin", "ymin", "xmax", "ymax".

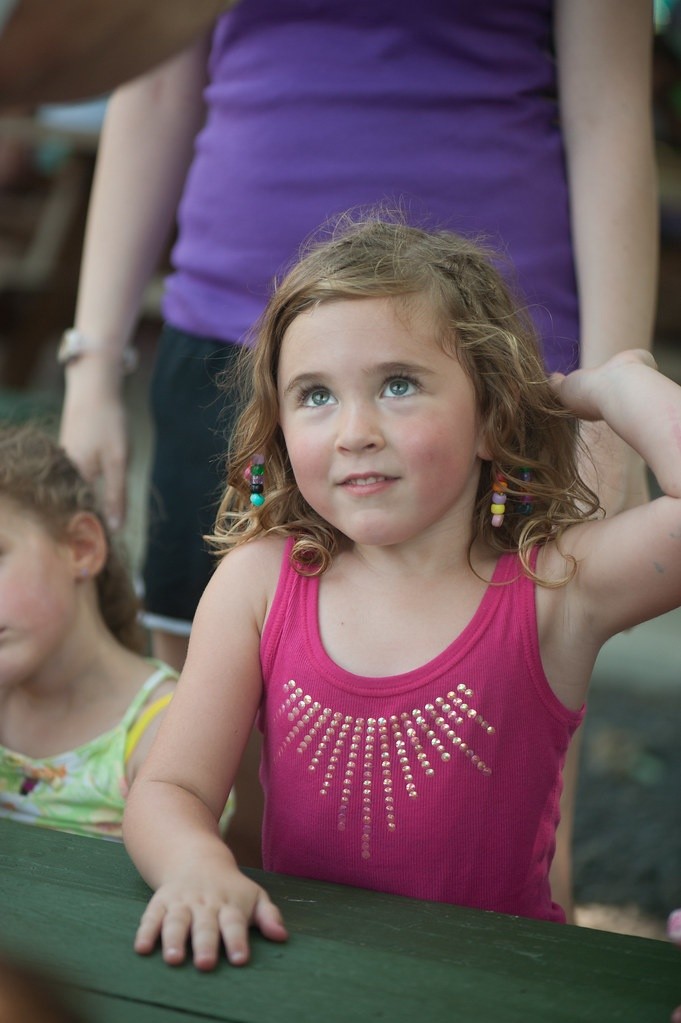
[{"xmin": 1, "ymin": 818, "xmax": 681, "ymax": 1022}]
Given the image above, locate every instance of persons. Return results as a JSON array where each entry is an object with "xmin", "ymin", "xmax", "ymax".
[
  {"xmin": 0, "ymin": 0, "xmax": 661, "ymax": 919},
  {"xmin": 120, "ymin": 204, "xmax": 681, "ymax": 972}
]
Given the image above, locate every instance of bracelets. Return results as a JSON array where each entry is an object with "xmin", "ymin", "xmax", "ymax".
[{"xmin": 54, "ymin": 330, "xmax": 137, "ymax": 375}]
